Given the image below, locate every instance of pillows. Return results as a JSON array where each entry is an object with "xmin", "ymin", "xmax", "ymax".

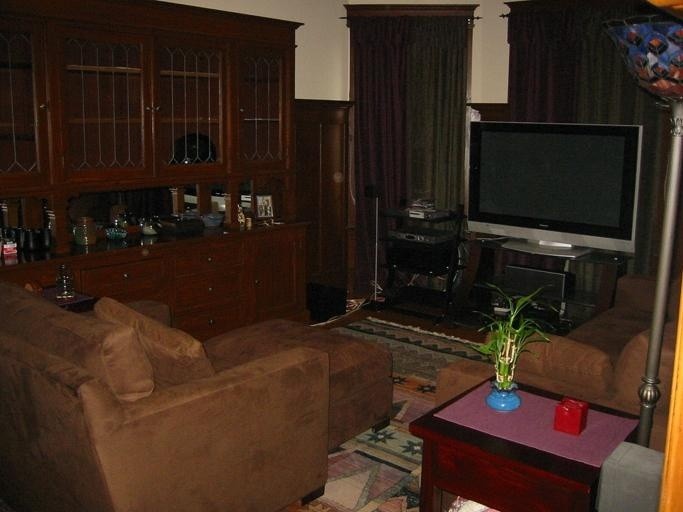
[{"xmin": 94, "ymin": 296, "xmax": 216, "ymax": 389}]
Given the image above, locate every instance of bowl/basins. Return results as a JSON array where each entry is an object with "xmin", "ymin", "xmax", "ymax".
[
  {"xmin": 103, "ymin": 227, "xmax": 127, "ymax": 240},
  {"xmin": 201, "ymin": 214, "xmax": 224, "ymax": 228}
]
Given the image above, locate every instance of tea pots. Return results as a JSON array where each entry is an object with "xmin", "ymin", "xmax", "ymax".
[{"xmin": 138, "ymin": 213, "xmax": 162, "ymax": 235}]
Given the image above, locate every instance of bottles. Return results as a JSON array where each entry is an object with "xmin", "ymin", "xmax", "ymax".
[
  {"xmin": 72, "ymin": 216, "xmax": 97, "ymax": 247},
  {"xmin": 56, "ymin": 262, "xmax": 76, "ymax": 299}
]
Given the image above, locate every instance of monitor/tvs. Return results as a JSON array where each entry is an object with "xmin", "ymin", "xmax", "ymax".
[{"xmin": 466, "ymin": 120, "xmax": 644, "ymax": 258}]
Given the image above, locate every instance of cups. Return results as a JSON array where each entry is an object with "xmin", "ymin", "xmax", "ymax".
[
  {"xmin": 8, "ymin": 226, "xmax": 52, "ymax": 253},
  {"xmin": 245, "ymin": 218, "xmax": 252, "ymax": 230}
]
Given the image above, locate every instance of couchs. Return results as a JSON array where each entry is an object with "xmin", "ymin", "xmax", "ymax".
[{"xmin": 437, "ymin": 272, "xmax": 681, "ymax": 453}]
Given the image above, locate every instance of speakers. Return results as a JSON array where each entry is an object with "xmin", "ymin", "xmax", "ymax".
[{"xmin": 502, "ymin": 264, "xmax": 576, "ymax": 299}]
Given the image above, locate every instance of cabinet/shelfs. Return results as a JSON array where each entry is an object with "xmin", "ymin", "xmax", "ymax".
[
  {"xmin": 1, "ymin": 3, "xmax": 152, "ymax": 193},
  {"xmin": 152, "ymin": 0, "xmax": 305, "ymax": 189},
  {"xmin": 3, "ymin": 217, "xmax": 312, "ymax": 326}
]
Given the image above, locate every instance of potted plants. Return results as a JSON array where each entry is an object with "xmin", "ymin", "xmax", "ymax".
[{"xmin": 466, "ymin": 279, "xmax": 560, "ymax": 413}]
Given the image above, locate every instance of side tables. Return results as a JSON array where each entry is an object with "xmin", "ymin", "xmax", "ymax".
[{"xmin": 409, "ymin": 375, "xmax": 640, "ymax": 512}]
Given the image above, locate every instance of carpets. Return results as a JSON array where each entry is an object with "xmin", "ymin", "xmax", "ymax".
[{"xmin": 320, "ymin": 317, "xmax": 502, "ymax": 511}]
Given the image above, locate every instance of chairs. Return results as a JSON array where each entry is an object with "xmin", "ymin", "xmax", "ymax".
[{"xmin": 1, "ymin": 283, "xmax": 395, "ymax": 512}]
